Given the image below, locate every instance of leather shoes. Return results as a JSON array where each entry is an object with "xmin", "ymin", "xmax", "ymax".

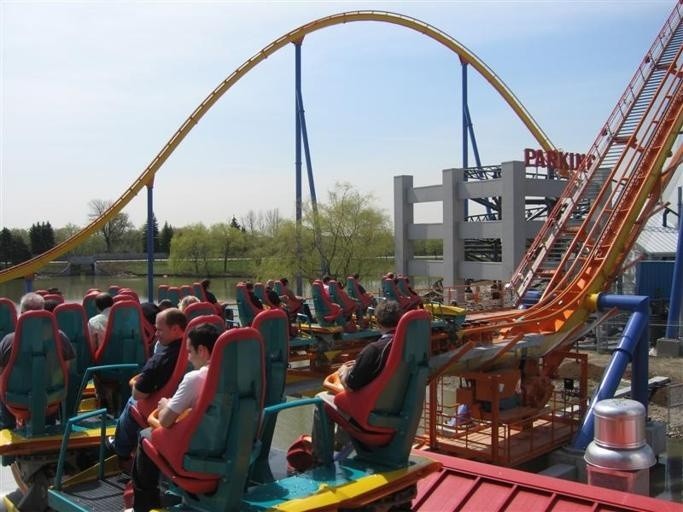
[{"xmin": 105, "ymin": 436, "xmax": 129, "ymax": 459}]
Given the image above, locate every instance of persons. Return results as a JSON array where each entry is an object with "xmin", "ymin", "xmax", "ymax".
[
  {"xmin": 386, "ymin": 272, "xmax": 424, "ymax": 310},
  {"xmin": 489, "ymin": 207, "xmax": 540, "ymax": 221},
  {"xmin": 153, "ymin": 295, "xmax": 200, "ymax": 353},
  {"xmin": 243, "ymin": 273, "xmax": 377, "ymax": 336},
  {"xmin": 202, "ymin": 279, "xmax": 223, "ymax": 314},
  {"xmin": 141, "ymin": 300, "xmax": 173, "ymax": 325},
  {"xmin": 434, "ymin": 278, "xmax": 444, "ymax": 290},
  {"xmin": 0, "ymin": 293, "xmax": 76, "ymax": 431},
  {"xmin": 465, "ymin": 278, "xmax": 473, "ymax": 292},
  {"xmin": 449, "ymin": 301, "xmax": 457, "ymax": 307},
  {"xmin": 334, "ymin": 301, "xmax": 403, "ymax": 451},
  {"xmin": 104, "ymin": 307, "xmax": 194, "ymax": 459},
  {"xmin": 491, "ymin": 279, "xmax": 503, "ymax": 299},
  {"xmin": 88, "ymin": 292, "xmax": 114, "ymax": 414},
  {"xmin": 443, "ymin": 360, "xmax": 525, "ymax": 426},
  {"xmin": 125, "ymin": 323, "xmax": 222, "ymax": 512}
]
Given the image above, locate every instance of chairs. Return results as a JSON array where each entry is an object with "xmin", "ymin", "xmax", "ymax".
[
  {"xmin": 140, "ymin": 328, "xmax": 265, "ymax": 511},
  {"xmin": 96, "ymin": 301, "xmax": 146, "ymax": 419},
  {"xmin": 251, "ymin": 310, "xmax": 290, "ymax": 482},
  {"xmin": 128, "ymin": 315, "xmax": 223, "ymax": 429},
  {"xmin": 54, "ymin": 303, "xmax": 96, "ymax": 422},
  {"xmin": 1, "ymin": 310, "xmax": 68, "ymax": 437},
  {"xmin": 1, "ymin": 278, "xmax": 425, "ymax": 335},
  {"xmin": 315, "ymin": 309, "xmax": 431, "ymax": 469}
]
